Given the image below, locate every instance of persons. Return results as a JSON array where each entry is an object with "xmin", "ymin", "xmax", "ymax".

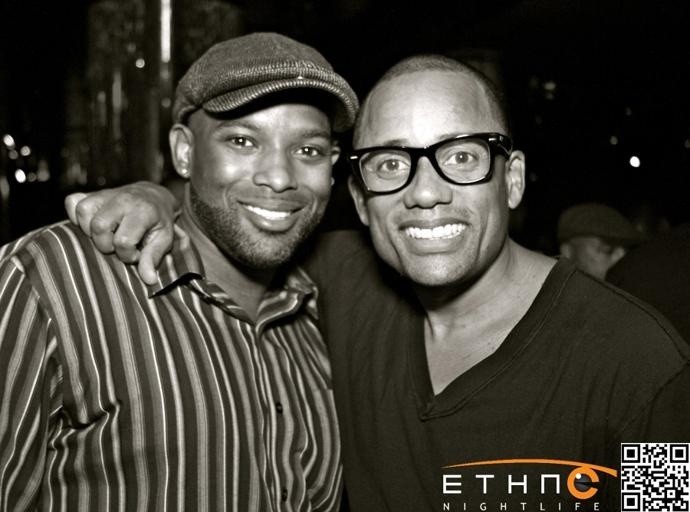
[{"xmin": 1, "ymin": 33, "xmax": 690, "ymax": 511}]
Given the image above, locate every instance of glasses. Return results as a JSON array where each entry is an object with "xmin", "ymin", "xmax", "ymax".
[{"xmin": 350, "ymin": 132, "xmax": 514, "ymax": 195}]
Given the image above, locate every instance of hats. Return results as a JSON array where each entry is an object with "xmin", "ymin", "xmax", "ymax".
[{"xmin": 172, "ymin": 30, "xmax": 360, "ymax": 134}]
